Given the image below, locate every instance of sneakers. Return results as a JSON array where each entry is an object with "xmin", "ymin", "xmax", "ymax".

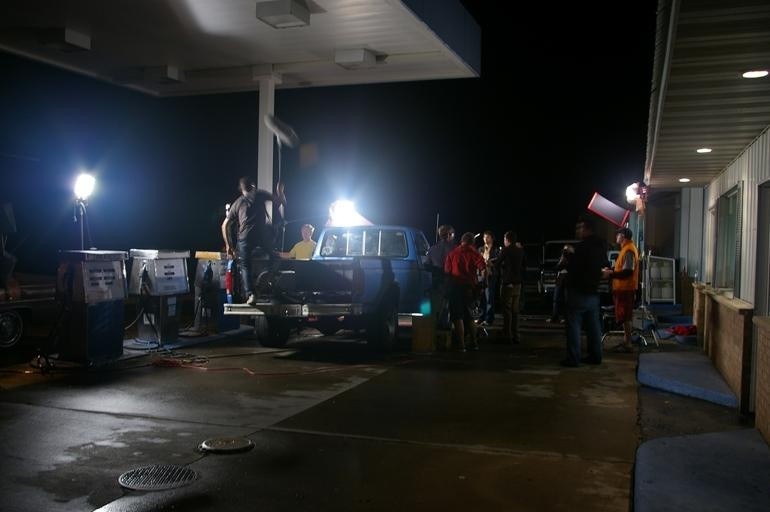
[
  {"xmin": 246, "ymin": 295, "xmax": 256, "ymax": 304},
  {"xmin": 559, "ymin": 358, "xmax": 580, "ymax": 368},
  {"xmin": 471, "ymin": 344, "xmax": 480, "ymax": 350},
  {"xmin": 582, "ymin": 357, "xmax": 603, "ymax": 365},
  {"xmin": 457, "ymin": 345, "xmax": 467, "ymax": 352},
  {"xmin": 611, "ymin": 344, "xmax": 633, "ymax": 353}
]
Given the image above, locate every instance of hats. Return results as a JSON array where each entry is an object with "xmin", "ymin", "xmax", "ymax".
[{"xmin": 616, "ymin": 228, "xmax": 632, "ymax": 237}]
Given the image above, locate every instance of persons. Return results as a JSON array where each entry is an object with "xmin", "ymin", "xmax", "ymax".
[
  {"xmin": 546, "ymin": 243, "xmax": 575, "ymax": 325},
  {"xmin": 602, "ymin": 228, "xmax": 639, "ymax": 353},
  {"xmin": 221, "ymin": 176, "xmax": 286, "ymax": 305},
  {"xmin": 423, "ymin": 225, "xmax": 526, "ymax": 352},
  {"xmin": 289, "ymin": 224, "xmax": 317, "ymax": 260},
  {"xmin": 540, "ymin": 216, "xmax": 604, "ymax": 367}
]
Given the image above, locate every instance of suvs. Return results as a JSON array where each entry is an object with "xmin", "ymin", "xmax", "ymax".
[{"xmin": 537, "ymin": 240, "xmax": 610, "ymax": 295}]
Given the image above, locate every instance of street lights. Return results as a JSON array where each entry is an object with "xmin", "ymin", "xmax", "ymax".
[
  {"xmin": 625, "ymin": 182, "xmax": 649, "ymax": 330},
  {"xmin": 68, "ymin": 172, "xmax": 98, "ymax": 250}
]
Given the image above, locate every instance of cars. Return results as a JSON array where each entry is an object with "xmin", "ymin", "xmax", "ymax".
[{"xmin": 1, "ymin": 262, "xmax": 71, "ymax": 348}]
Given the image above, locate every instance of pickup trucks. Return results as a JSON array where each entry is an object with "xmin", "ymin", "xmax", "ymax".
[{"xmin": 223, "ymin": 224, "xmax": 433, "ymax": 357}]
